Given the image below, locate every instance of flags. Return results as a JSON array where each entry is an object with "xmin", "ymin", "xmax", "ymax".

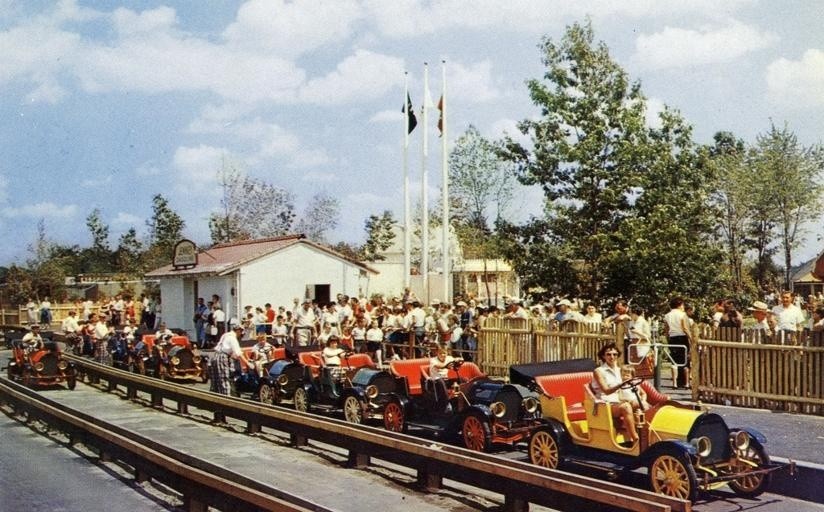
[
  {"xmin": 436, "ymin": 90, "xmax": 449, "ymax": 140},
  {"xmin": 417, "ymin": 80, "xmax": 436, "ymax": 125},
  {"xmin": 401, "ymin": 88, "xmax": 417, "ymax": 134}
]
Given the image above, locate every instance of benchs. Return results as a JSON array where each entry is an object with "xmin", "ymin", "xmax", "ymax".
[{"xmin": 140, "ymin": 331, "xmax": 661, "ymax": 422}]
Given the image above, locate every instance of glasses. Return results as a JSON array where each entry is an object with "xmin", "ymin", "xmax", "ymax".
[{"xmin": 606, "ymin": 353, "xmax": 618, "ymax": 356}]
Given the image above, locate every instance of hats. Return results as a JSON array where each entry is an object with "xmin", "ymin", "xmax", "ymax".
[
  {"xmin": 99, "ymin": 314, "xmax": 107, "ymax": 317},
  {"xmin": 457, "ymin": 301, "xmax": 468, "ymax": 308},
  {"xmin": 233, "ymin": 324, "xmax": 246, "ymax": 331},
  {"xmin": 746, "ymin": 300, "xmax": 769, "ymax": 314},
  {"xmin": 556, "ymin": 299, "xmax": 571, "ymax": 307},
  {"xmin": 431, "ymin": 298, "xmax": 440, "ymax": 305}
]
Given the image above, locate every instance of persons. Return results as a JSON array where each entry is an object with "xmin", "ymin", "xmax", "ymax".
[
  {"xmin": 22, "ymin": 294, "xmax": 174, "ymax": 374},
  {"xmin": 617, "ymin": 364, "xmax": 650, "ymax": 416},
  {"xmin": 591, "ymin": 342, "xmax": 642, "ymax": 442},
  {"xmin": 429, "ymin": 347, "xmax": 465, "ymax": 412},
  {"xmin": 194, "ymin": 292, "xmax": 823, "ymax": 395}
]
